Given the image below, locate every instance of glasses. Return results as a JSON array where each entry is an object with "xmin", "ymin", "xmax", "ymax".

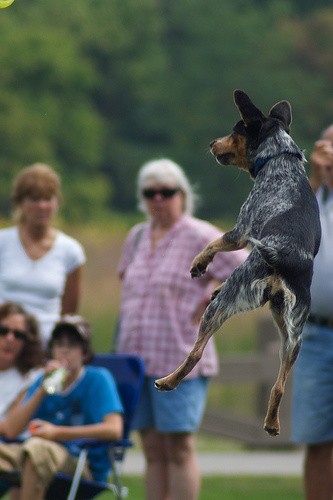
[
  {"xmin": 0, "ymin": 327, "xmax": 27, "ymax": 339},
  {"xmin": 142, "ymin": 185, "xmax": 181, "ymax": 198}
]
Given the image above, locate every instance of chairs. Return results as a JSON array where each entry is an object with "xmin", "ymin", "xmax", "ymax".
[{"xmin": 0, "ymin": 353, "xmax": 144, "ymax": 500}]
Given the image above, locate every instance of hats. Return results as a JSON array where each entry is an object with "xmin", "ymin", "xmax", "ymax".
[{"xmin": 50, "ymin": 313, "xmax": 92, "ymax": 347}]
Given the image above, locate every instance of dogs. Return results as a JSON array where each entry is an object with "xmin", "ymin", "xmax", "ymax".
[{"xmin": 154, "ymin": 88, "xmax": 322, "ymax": 437}]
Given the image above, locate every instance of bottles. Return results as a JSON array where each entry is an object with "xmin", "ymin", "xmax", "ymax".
[{"xmin": 44, "ymin": 358, "xmax": 68, "ymax": 394}]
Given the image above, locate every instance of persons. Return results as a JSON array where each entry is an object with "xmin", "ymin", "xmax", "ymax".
[
  {"xmin": 0, "ymin": 162, "xmax": 124, "ymax": 500},
  {"xmin": 289, "ymin": 125, "xmax": 333, "ymax": 500},
  {"xmin": 116, "ymin": 157, "xmax": 250, "ymax": 500}
]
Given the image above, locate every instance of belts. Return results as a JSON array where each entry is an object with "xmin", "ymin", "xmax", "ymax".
[{"xmin": 307, "ymin": 314, "xmax": 333, "ymax": 330}]
